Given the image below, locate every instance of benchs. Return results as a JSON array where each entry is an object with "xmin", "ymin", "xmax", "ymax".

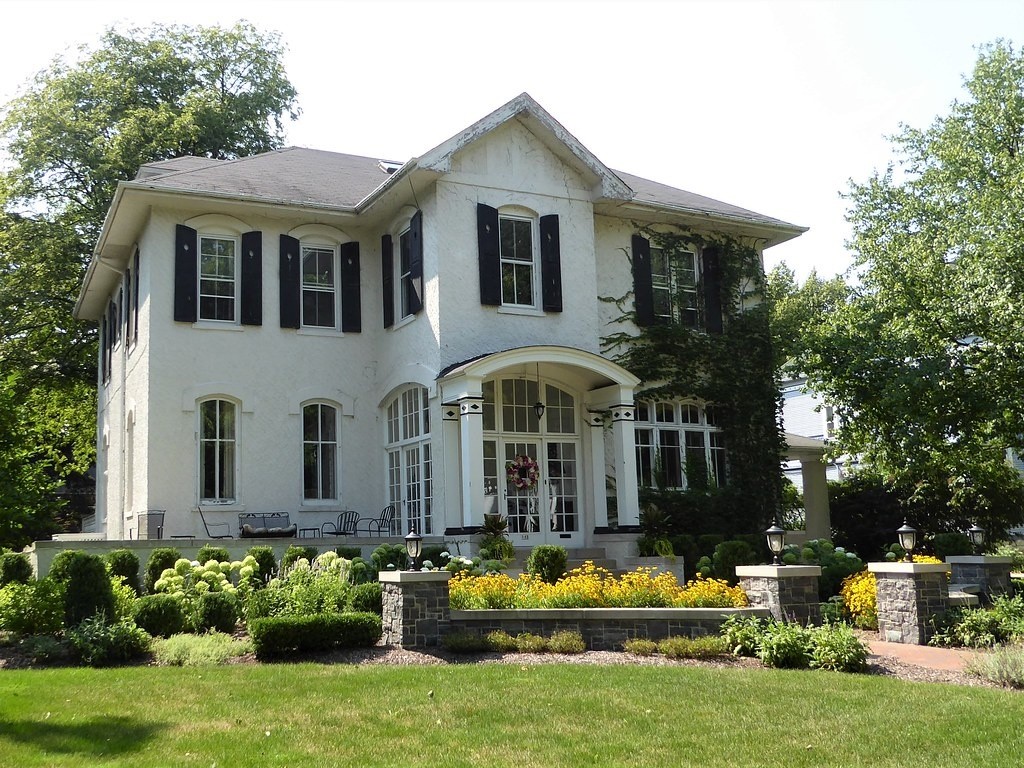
[{"xmin": 237, "ymin": 512, "xmax": 297, "ymax": 538}]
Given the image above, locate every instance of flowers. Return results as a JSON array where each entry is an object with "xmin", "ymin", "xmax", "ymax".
[{"xmin": 508, "ymin": 456, "xmax": 541, "ymax": 490}]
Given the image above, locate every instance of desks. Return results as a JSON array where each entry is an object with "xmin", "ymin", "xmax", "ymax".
[
  {"xmin": 299, "ymin": 527, "xmax": 320, "ymax": 539},
  {"xmin": 170, "ymin": 536, "xmax": 196, "ymax": 539}
]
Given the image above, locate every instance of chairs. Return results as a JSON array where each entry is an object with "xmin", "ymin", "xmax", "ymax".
[
  {"xmin": 321, "ymin": 511, "xmax": 360, "ymax": 538},
  {"xmin": 353, "ymin": 505, "xmax": 395, "ymax": 536},
  {"xmin": 199, "ymin": 507, "xmax": 234, "ymax": 539}
]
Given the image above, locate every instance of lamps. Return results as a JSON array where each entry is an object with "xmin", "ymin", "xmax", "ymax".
[
  {"xmin": 896, "ymin": 517, "xmax": 918, "ymax": 563},
  {"xmin": 405, "ymin": 527, "xmax": 422, "ymax": 571},
  {"xmin": 763, "ymin": 516, "xmax": 790, "ymax": 568},
  {"xmin": 968, "ymin": 522, "xmax": 986, "ymax": 555},
  {"xmin": 533, "ymin": 362, "xmax": 544, "ymax": 419}
]
